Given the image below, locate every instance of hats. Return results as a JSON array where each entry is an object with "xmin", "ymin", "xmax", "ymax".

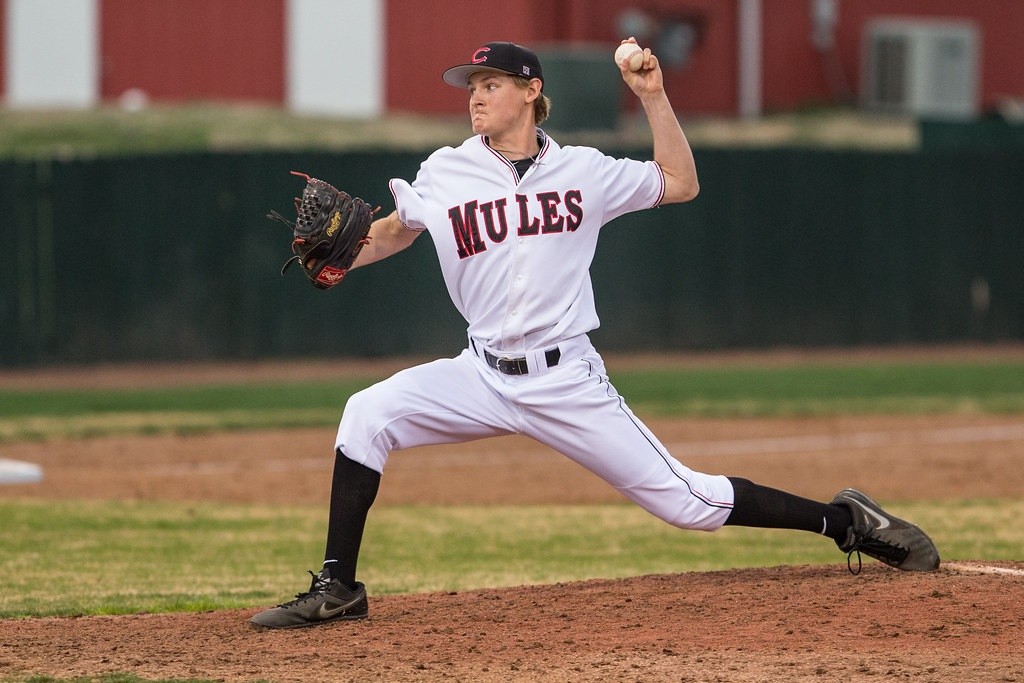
[{"xmin": 441, "ymin": 42, "xmax": 544, "ymax": 88}]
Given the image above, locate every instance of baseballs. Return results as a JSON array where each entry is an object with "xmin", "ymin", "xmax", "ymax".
[{"xmin": 614, "ymin": 42, "xmax": 643, "ymax": 70}]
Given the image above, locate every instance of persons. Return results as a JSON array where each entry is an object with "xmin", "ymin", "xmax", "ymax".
[{"xmin": 251, "ymin": 36, "xmax": 940, "ymax": 631}]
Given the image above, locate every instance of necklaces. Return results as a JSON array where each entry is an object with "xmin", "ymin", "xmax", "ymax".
[{"xmin": 497, "ymin": 136, "xmax": 545, "ymax": 168}]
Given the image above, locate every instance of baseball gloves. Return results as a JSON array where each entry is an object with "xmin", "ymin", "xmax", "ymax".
[{"xmin": 266, "ymin": 169, "xmax": 382, "ymax": 291}]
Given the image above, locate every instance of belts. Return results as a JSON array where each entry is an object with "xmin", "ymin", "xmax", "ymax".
[{"xmin": 470, "ymin": 337, "xmax": 560, "ymax": 375}]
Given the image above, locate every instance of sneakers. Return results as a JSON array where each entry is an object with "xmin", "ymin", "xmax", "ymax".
[
  {"xmin": 829, "ymin": 488, "xmax": 940, "ymax": 575},
  {"xmin": 249, "ymin": 568, "xmax": 369, "ymax": 628}
]
